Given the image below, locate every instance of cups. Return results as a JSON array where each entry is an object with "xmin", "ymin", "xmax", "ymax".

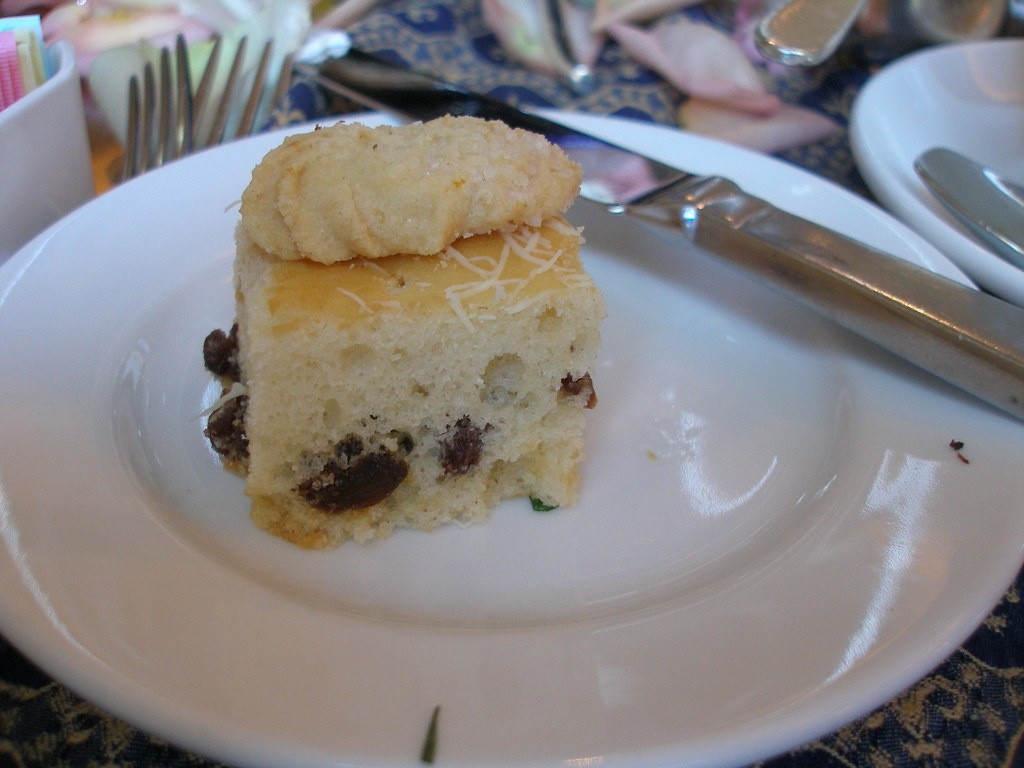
[{"xmin": 0, "ymin": 39, "xmax": 95, "ymax": 264}]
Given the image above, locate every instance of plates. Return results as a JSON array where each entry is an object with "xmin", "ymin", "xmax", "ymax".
[
  {"xmin": 852, "ymin": 40, "xmax": 1024, "ymax": 306},
  {"xmin": 0, "ymin": 110, "xmax": 1024, "ymax": 768}
]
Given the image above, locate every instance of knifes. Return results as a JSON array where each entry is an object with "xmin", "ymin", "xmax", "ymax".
[
  {"xmin": 298, "ymin": 41, "xmax": 1024, "ymax": 417},
  {"xmin": 914, "ymin": 150, "xmax": 1024, "ymax": 265}
]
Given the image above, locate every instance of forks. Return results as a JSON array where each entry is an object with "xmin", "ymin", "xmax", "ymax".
[
  {"xmin": 194, "ymin": 36, "xmax": 292, "ymax": 148},
  {"xmin": 121, "ymin": 36, "xmax": 194, "ymax": 179}
]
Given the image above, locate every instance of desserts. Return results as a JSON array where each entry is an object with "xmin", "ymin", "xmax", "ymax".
[{"xmin": 203, "ymin": 113, "xmax": 600, "ymax": 551}]
